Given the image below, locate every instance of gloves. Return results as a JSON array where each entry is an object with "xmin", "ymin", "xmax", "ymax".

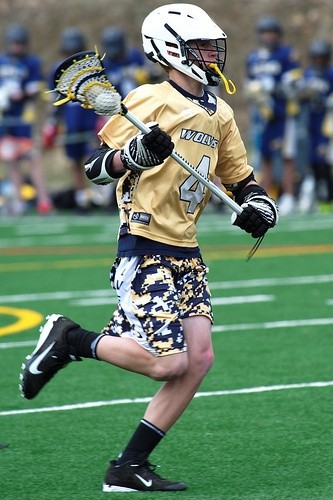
[
  {"xmin": 230, "ymin": 184, "xmax": 279, "ymax": 238},
  {"xmin": 120, "ymin": 121, "xmax": 175, "ymax": 170}
]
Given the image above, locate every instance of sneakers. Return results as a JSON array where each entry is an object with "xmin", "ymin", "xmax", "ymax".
[
  {"xmin": 102, "ymin": 459, "xmax": 187, "ymax": 492},
  {"xmin": 18, "ymin": 314, "xmax": 82, "ymax": 400}
]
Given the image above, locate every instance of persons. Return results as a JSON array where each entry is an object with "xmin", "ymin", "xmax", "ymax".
[
  {"xmin": 18, "ymin": 4, "xmax": 278, "ymax": 494},
  {"xmin": 0, "ymin": 18, "xmax": 333, "ymax": 217}
]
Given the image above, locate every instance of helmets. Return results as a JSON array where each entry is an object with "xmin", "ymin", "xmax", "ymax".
[
  {"xmin": 101, "ymin": 27, "xmax": 126, "ymax": 54},
  {"xmin": 307, "ymin": 40, "xmax": 332, "ymax": 56},
  {"xmin": 5, "ymin": 25, "xmax": 30, "ymax": 45},
  {"xmin": 141, "ymin": 4, "xmax": 227, "ymax": 86},
  {"xmin": 60, "ymin": 28, "xmax": 87, "ymax": 54},
  {"xmin": 257, "ymin": 16, "xmax": 283, "ymax": 34}
]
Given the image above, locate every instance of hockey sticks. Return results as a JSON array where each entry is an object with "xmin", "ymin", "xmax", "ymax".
[{"xmin": 44, "ymin": 44, "xmax": 243, "ymax": 214}]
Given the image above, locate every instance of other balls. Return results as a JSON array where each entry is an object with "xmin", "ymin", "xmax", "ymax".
[{"xmin": 94, "ymin": 94, "xmax": 114, "ymax": 113}]
